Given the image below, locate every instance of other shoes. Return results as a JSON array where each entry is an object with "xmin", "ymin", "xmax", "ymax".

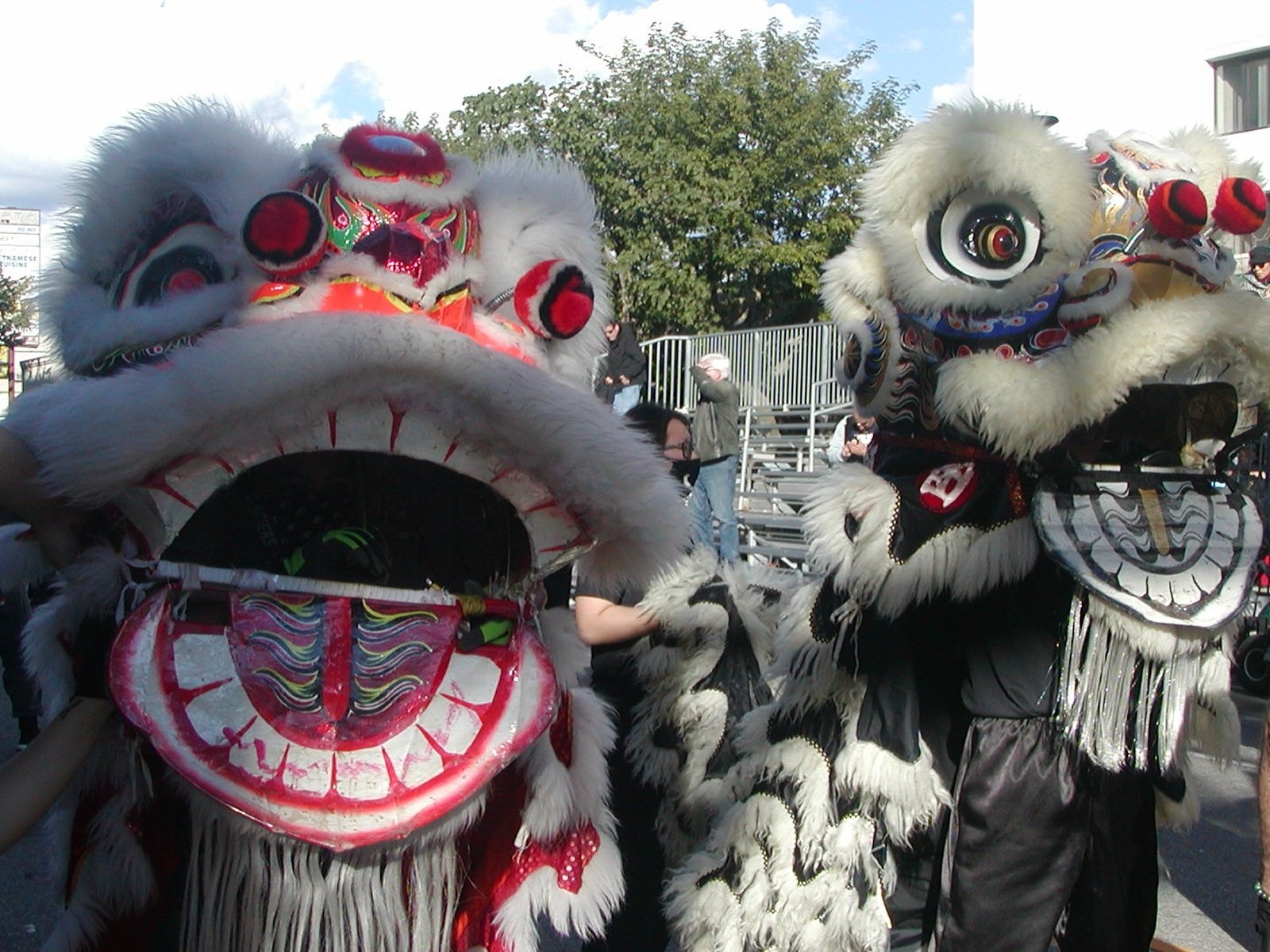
[{"xmin": 1254, "ymin": 882, "xmax": 1270, "ymax": 936}]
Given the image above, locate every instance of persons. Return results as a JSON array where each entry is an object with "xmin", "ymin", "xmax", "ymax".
[
  {"xmin": 601, "ymin": 318, "xmax": 646, "ymax": 417},
  {"xmin": 1246, "ymin": 702, "xmax": 1270, "ymax": 952},
  {"xmin": 686, "ymin": 353, "xmax": 743, "ymax": 566},
  {"xmin": 1247, "ymin": 246, "xmax": 1270, "ymax": 299},
  {"xmin": 825, "ymin": 400, "xmax": 877, "ymax": 468},
  {"xmin": 574, "ymin": 400, "xmax": 702, "ymax": 941},
  {"xmin": 841, "ymin": 598, "xmax": 971, "ymax": 952},
  {"xmin": 929, "ymin": 563, "xmax": 1161, "ymax": 952}
]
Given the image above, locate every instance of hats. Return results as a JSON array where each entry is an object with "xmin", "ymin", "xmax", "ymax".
[{"xmin": 1249, "ymin": 246, "xmax": 1270, "ymax": 263}]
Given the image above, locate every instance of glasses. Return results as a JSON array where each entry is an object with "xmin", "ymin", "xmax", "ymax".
[
  {"xmin": 663, "ymin": 442, "xmax": 694, "ymax": 459},
  {"xmin": 607, "ymin": 324, "xmax": 616, "ymax": 334}
]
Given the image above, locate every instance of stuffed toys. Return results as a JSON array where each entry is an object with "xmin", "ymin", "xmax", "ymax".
[
  {"xmin": 0, "ymin": 94, "xmax": 777, "ymax": 952},
  {"xmin": 655, "ymin": 97, "xmax": 1270, "ymax": 952}
]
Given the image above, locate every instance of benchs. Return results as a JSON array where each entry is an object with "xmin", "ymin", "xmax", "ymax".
[{"xmin": 711, "ymin": 436, "xmax": 834, "ymax": 581}]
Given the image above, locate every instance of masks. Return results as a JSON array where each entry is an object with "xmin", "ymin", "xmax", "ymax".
[{"xmin": 668, "ymin": 458, "xmax": 700, "ymax": 497}]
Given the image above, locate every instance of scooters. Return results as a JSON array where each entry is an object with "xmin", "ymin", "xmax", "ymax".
[{"xmin": 1233, "ymin": 598, "xmax": 1270, "ymax": 694}]
